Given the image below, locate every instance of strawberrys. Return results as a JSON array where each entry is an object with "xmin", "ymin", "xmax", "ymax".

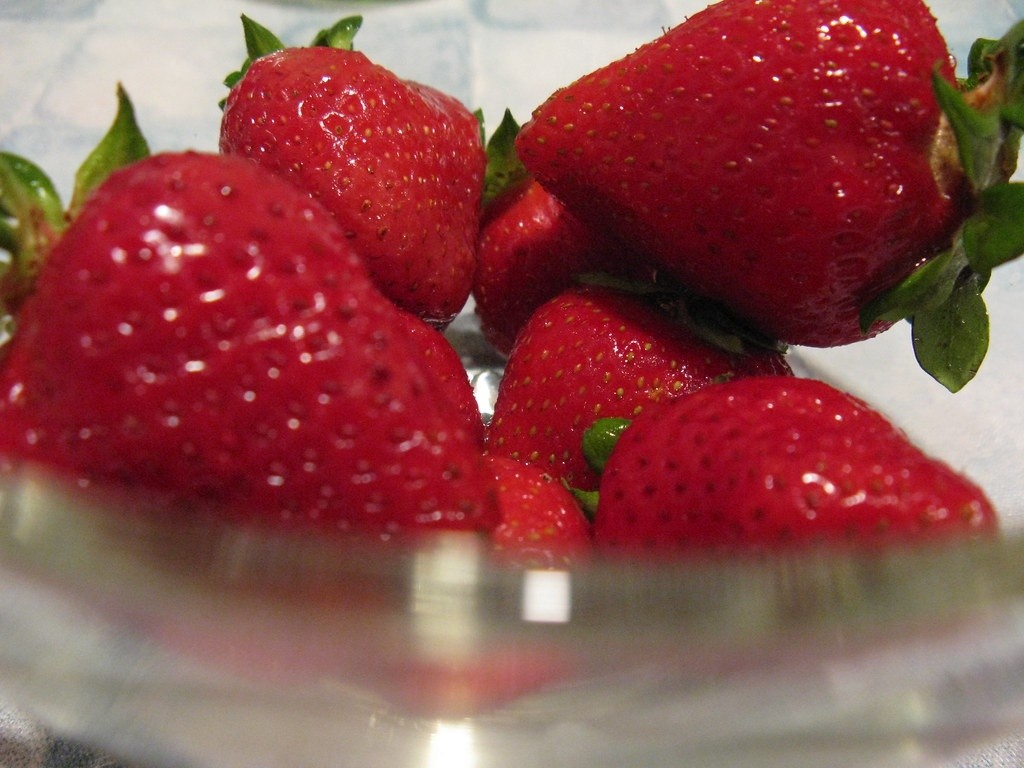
[{"xmin": 0, "ymin": 0, "xmax": 1024, "ymax": 716}]
